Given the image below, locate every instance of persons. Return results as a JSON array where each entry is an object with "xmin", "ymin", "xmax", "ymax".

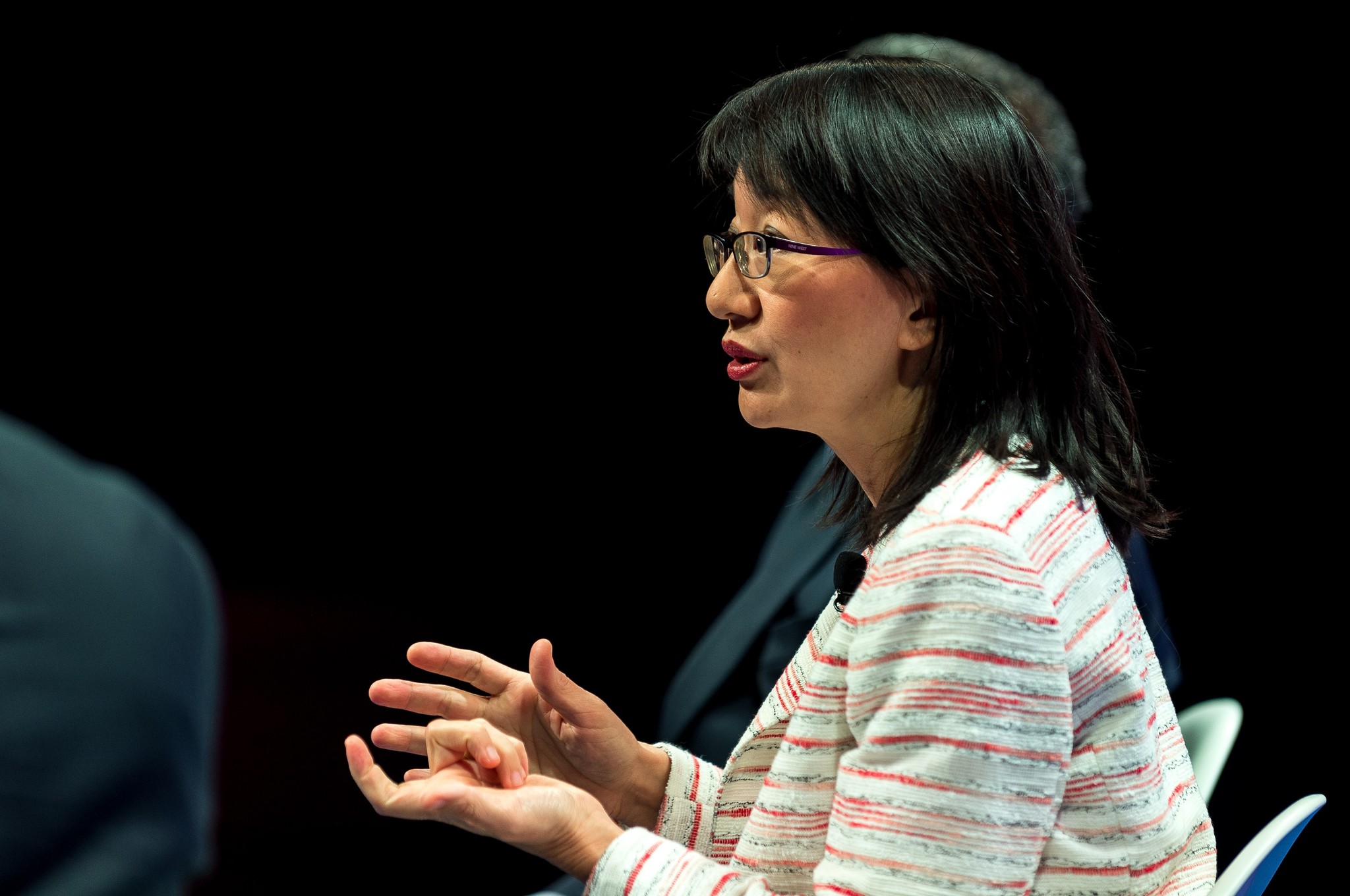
[
  {"xmin": 0, "ymin": 407, "xmax": 228, "ymax": 895},
  {"xmin": 522, "ymin": 31, "xmax": 1185, "ymax": 895},
  {"xmin": 335, "ymin": 65, "xmax": 1229, "ymax": 895}
]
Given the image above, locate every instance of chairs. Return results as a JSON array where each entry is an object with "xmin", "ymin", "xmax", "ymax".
[
  {"xmin": 1207, "ymin": 793, "xmax": 1326, "ymax": 896},
  {"xmin": 1178, "ymin": 698, "xmax": 1243, "ymax": 805}
]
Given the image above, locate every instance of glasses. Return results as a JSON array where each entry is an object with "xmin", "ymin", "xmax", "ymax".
[{"xmin": 702, "ymin": 233, "xmax": 862, "ymax": 278}]
